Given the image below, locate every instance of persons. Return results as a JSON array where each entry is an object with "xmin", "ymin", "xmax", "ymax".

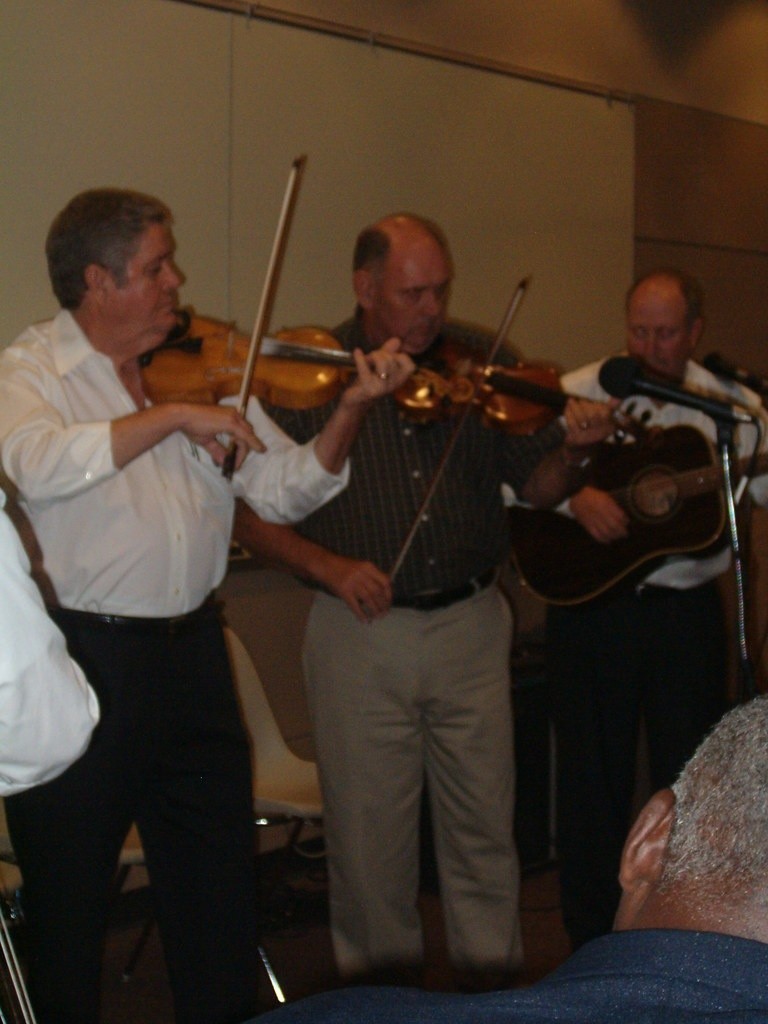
[
  {"xmin": 496, "ymin": 267, "xmax": 768, "ymax": 950},
  {"xmin": 0, "ymin": 184, "xmax": 421, "ymax": 1021},
  {"xmin": 222, "ymin": 213, "xmax": 622, "ymax": 962},
  {"xmin": 0, "ymin": 487, "xmax": 102, "ymax": 797},
  {"xmin": 250, "ymin": 695, "xmax": 767, "ymax": 1023}
]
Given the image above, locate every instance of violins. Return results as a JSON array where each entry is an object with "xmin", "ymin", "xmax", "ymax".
[
  {"xmin": 140, "ymin": 303, "xmax": 456, "ymax": 415},
  {"xmin": 430, "ymin": 339, "xmax": 666, "ymax": 448}
]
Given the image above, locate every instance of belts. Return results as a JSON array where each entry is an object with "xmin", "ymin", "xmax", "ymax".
[
  {"xmin": 391, "ymin": 572, "xmax": 496, "ymax": 609},
  {"xmin": 68, "ymin": 597, "xmax": 215, "ymax": 625}
]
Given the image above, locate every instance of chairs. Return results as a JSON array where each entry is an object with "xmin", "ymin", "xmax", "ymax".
[
  {"xmin": 117, "ymin": 821, "xmax": 159, "ymax": 980},
  {"xmin": 214, "ymin": 568, "xmax": 329, "ymax": 824},
  {"xmin": 0, "ymin": 860, "xmax": 37, "ymax": 1024}
]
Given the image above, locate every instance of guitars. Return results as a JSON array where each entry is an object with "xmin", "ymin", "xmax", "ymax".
[{"xmin": 507, "ymin": 424, "xmax": 766, "ymax": 607}]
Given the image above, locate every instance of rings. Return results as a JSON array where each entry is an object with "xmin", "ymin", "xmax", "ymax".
[
  {"xmin": 376, "ymin": 372, "xmax": 391, "ymax": 380},
  {"xmin": 579, "ymin": 422, "xmax": 589, "ymax": 429}
]
